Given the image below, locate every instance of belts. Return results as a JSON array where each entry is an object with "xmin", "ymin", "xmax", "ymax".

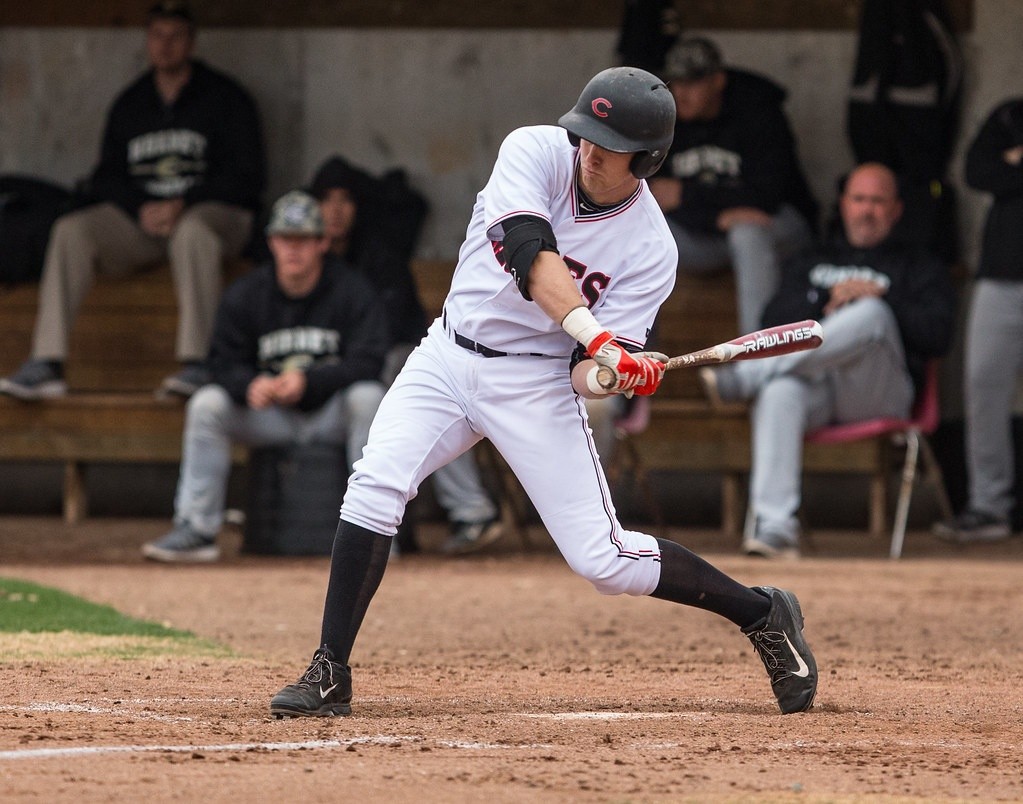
[{"xmin": 455, "ymin": 334, "xmax": 507, "ymax": 358}]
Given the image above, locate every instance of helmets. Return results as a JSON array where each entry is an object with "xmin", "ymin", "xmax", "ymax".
[
  {"xmin": 266, "ymin": 190, "xmax": 324, "ymax": 237},
  {"xmin": 664, "ymin": 34, "xmax": 720, "ymax": 78},
  {"xmin": 558, "ymin": 66, "xmax": 676, "ymax": 179}
]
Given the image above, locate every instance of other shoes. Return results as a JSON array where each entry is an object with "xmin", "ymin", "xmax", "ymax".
[
  {"xmin": 162, "ymin": 363, "xmax": 207, "ymax": 396},
  {"xmin": 745, "ymin": 529, "xmax": 801, "ymax": 558},
  {"xmin": 141, "ymin": 524, "xmax": 220, "ymax": 564},
  {"xmin": 439, "ymin": 518, "xmax": 506, "ymax": 559},
  {"xmin": 935, "ymin": 511, "xmax": 1010, "ymax": 544},
  {"xmin": 697, "ymin": 364, "xmax": 748, "ymax": 412},
  {"xmin": 0, "ymin": 362, "xmax": 68, "ymax": 399}
]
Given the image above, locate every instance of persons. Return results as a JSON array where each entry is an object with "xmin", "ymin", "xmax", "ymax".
[
  {"xmin": 700, "ymin": 165, "xmax": 930, "ymax": 557},
  {"xmin": 304, "ymin": 151, "xmax": 503, "ymax": 558},
  {"xmin": 0, "ymin": 6, "xmax": 270, "ymax": 401},
  {"xmin": 933, "ymin": 93, "xmax": 1023, "ymax": 541},
  {"xmin": 142, "ymin": 189, "xmax": 504, "ymax": 561},
  {"xmin": 269, "ymin": 66, "xmax": 819, "ymax": 718},
  {"xmin": 842, "ymin": 0, "xmax": 974, "ymax": 219},
  {"xmin": 646, "ymin": 38, "xmax": 822, "ymax": 332}
]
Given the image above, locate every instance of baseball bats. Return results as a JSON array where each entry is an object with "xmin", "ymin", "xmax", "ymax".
[{"xmin": 595, "ymin": 319, "xmax": 825, "ymax": 392}]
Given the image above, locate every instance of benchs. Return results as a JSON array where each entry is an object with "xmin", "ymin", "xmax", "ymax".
[{"xmin": 0, "ymin": 255, "xmax": 937, "ymax": 535}]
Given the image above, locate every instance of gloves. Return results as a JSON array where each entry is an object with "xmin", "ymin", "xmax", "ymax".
[
  {"xmin": 586, "ymin": 331, "xmax": 648, "ymax": 400},
  {"xmin": 586, "ymin": 351, "xmax": 669, "ymax": 397}
]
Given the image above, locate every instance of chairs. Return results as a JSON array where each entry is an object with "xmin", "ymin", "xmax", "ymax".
[{"xmin": 741, "ymin": 354, "xmax": 959, "ymax": 560}]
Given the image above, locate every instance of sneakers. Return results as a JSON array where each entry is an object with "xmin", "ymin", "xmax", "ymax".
[
  {"xmin": 740, "ymin": 586, "xmax": 818, "ymax": 714},
  {"xmin": 270, "ymin": 650, "xmax": 353, "ymax": 718}
]
